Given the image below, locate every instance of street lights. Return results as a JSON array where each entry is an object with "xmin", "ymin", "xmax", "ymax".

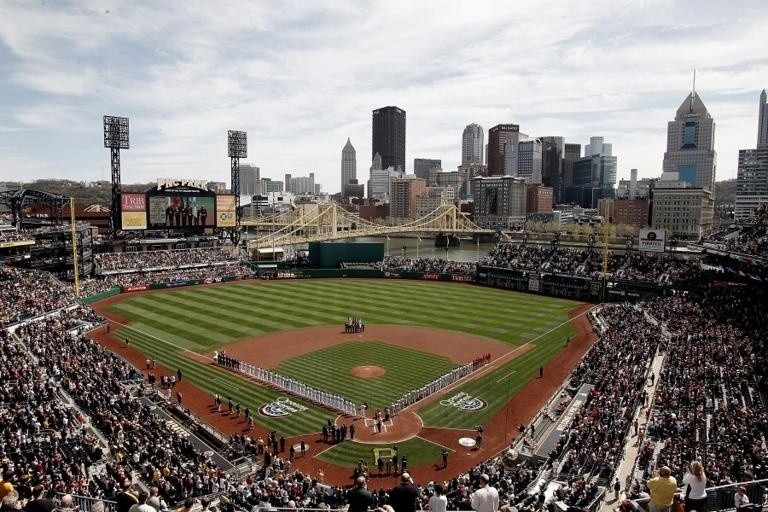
[
  {"xmin": 226, "ymin": 130, "xmax": 249, "ymax": 244},
  {"xmin": 103, "ymin": 115, "xmax": 130, "ymax": 238}
]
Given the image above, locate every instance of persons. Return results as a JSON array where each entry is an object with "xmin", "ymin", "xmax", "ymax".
[
  {"xmin": 1, "ymin": 224, "xmax": 767, "ymax": 511},
  {"xmin": 163, "ymin": 199, "xmax": 206, "ymax": 225}
]
